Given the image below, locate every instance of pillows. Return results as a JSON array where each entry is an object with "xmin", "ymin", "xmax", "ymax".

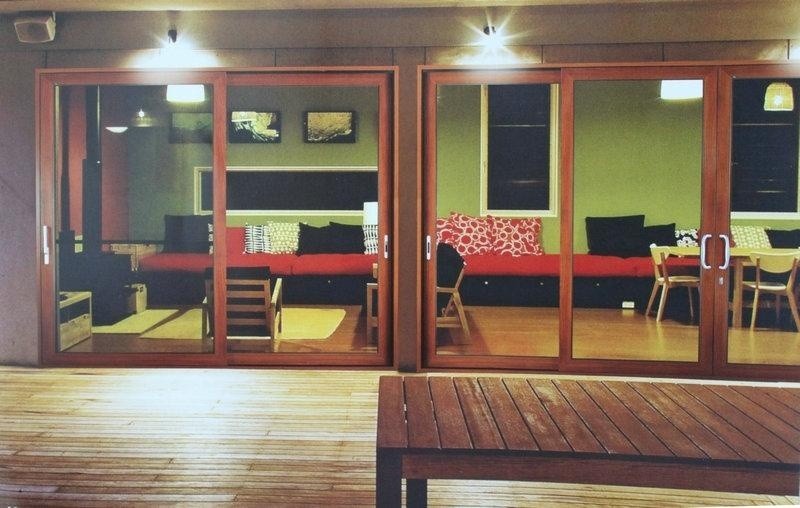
[
  {"xmin": 446, "ymin": 211, "xmax": 493, "ymax": 255},
  {"xmin": 163, "ymin": 214, "xmax": 217, "ymax": 253},
  {"xmin": 487, "ymin": 216, "xmax": 545, "ymax": 256},
  {"xmin": 645, "ymin": 222, "xmax": 679, "ymax": 255},
  {"xmin": 730, "ymin": 225, "xmax": 772, "ymax": 250},
  {"xmin": 299, "ymin": 221, "xmax": 328, "ymax": 252},
  {"xmin": 435, "ymin": 216, "xmax": 447, "ymax": 246},
  {"xmin": 225, "ymin": 225, "xmax": 245, "ymax": 255},
  {"xmin": 245, "ymin": 225, "xmax": 269, "ymax": 252},
  {"xmin": 267, "ymin": 220, "xmax": 304, "ymax": 255},
  {"xmin": 329, "ymin": 221, "xmax": 364, "ymax": 252},
  {"xmin": 766, "ymin": 229, "xmax": 800, "ymax": 249},
  {"xmin": 583, "ymin": 214, "xmax": 648, "ymax": 259},
  {"xmin": 674, "ymin": 228, "xmax": 700, "ymax": 247}
]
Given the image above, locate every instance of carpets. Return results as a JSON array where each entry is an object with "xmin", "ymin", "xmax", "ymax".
[{"xmin": 92, "ymin": 306, "xmax": 346, "ymax": 341}]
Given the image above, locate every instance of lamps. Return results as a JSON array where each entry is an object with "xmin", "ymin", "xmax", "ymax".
[
  {"xmin": 158, "ymin": 31, "xmax": 186, "ymax": 60},
  {"xmin": 763, "ymin": 81, "xmax": 795, "ymax": 112},
  {"xmin": 130, "ymin": 106, "xmax": 155, "ymax": 128},
  {"xmin": 165, "ymin": 84, "xmax": 206, "ymax": 106}
]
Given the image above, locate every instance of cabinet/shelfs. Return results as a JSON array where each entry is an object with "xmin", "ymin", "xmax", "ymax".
[{"xmin": 59, "ymin": 291, "xmax": 92, "ymax": 351}]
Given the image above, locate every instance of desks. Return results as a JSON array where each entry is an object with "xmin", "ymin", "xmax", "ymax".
[{"xmin": 374, "ymin": 375, "xmax": 800, "ymax": 508}]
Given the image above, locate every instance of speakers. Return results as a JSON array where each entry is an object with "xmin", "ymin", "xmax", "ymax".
[{"xmin": 13, "ymin": 15, "xmax": 55, "ymax": 43}]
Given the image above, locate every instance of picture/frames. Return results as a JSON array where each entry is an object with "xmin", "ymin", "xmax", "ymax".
[{"xmin": 168, "ymin": 109, "xmax": 359, "ymax": 145}]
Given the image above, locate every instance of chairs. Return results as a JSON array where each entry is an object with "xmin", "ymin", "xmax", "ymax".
[
  {"xmin": 198, "ymin": 245, "xmax": 470, "ymax": 353},
  {"xmin": 645, "ymin": 243, "xmax": 800, "ymax": 330}
]
